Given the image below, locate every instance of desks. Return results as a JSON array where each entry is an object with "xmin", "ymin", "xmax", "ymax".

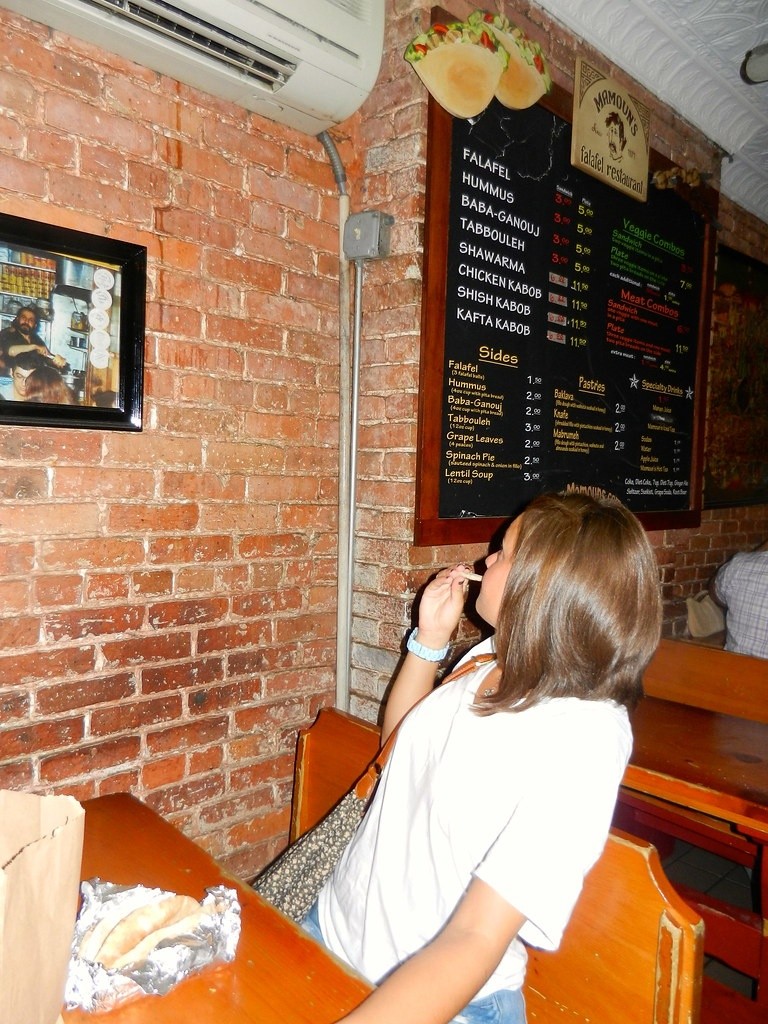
[
  {"xmin": 617, "ymin": 693, "xmax": 768, "ymax": 1024},
  {"xmin": 60, "ymin": 790, "xmax": 379, "ymax": 1024}
]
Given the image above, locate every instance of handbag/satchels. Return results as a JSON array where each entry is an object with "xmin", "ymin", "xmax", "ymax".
[
  {"xmin": 0, "ymin": 789, "xmax": 86, "ymax": 1023},
  {"xmin": 685, "ymin": 590, "xmax": 726, "ymax": 645},
  {"xmin": 250, "ymin": 655, "xmax": 498, "ymax": 925}
]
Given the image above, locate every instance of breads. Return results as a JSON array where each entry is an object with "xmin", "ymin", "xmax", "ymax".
[{"xmin": 76, "ymin": 890, "xmax": 212, "ymax": 970}]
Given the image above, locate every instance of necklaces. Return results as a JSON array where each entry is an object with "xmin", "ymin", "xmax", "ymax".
[{"xmin": 484, "ymin": 675, "xmax": 502, "ymax": 697}]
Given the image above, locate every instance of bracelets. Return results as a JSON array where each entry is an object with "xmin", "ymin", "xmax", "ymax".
[{"xmin": 406, "ymin": 627, "xmax": 450, "ymax": 662}]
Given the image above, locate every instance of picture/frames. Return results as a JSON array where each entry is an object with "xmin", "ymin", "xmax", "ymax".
[{"xmin": 0, "ymin": 212, "xmax": 147, "ymax": 432}]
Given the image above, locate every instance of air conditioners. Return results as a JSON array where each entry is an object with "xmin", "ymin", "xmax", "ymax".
[{"xmin": 0, "ymin": 0, "xmax": 386, "ymax": 138}]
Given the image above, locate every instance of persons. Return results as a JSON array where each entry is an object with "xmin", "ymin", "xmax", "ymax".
[
  {"xmin": 299, "ymin": 489, "xmax": 767, "ymax": 1024},
  {"xmin": 0, "ymin": 305, "xmax": 82, "ymax": 405}
]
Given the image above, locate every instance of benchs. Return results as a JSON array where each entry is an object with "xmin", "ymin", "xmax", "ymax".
[
  {"xmin": 289, "ymin": 705, "xmax": 705, "ymax": 1024},
  {"xmin": 642, "ymin": 639, "xmax": 768, "ymax": 723}
]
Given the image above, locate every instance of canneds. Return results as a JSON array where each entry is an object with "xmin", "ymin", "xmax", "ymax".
[
  {"xmin": 0, "ymin": 248, "xmax": 57, "ymax": 300},
  {"xmin": 71, "ymin": 311, "xmax": 85, "ymax": 330}
]
